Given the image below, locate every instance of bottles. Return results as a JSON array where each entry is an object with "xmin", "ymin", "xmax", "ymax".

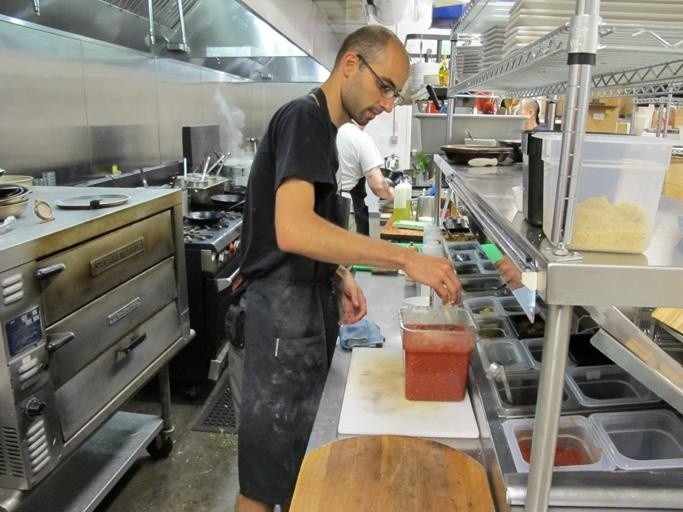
[
  {"xmin": 404, "ymin": 241, "xmax": 418, "ymax": 287},
  {"xmin": 438, "ymin": 55, "xmax": 449, "ymax": 88},
  {"xmin": 393, "ymin": 177, "xmax": 412, "ymax": 222}
]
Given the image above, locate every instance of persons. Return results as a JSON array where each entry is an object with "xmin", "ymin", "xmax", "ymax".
[
  {"xmin": 519, "ymin": 99, "xmax": 548, "ymax": 134},
  {"xmin": 333, "ymin": 118, "xmax": 395, "ymax": 238},
  {"xmin": 230, "ymin": 25, "xmax": 464, "ymax": 510}
]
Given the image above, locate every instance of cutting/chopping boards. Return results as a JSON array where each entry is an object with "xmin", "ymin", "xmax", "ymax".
[
  {"xmin": 288, "ymin": 435, "xmax": 497, "ymax": 512},
  {"xmin": 336, "ymin": 346, "xmax": 481, "ymax": 439},
  {"xmin": 379, "ymin": 214, "xmax": 423, "ymax": 240}
]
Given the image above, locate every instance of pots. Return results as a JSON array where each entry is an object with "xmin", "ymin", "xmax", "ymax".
[
  {"xmin": 183, "ymin": 209, "xmax": 225, "ymax": 224},
  {"xmin": 210, "ymin": 194, "xmax": 241, "ymax": 206},
  {"xmin": 175, "ymin": 173, "xmax": 217, "ymax": 188},
  {"xmin": 218, "ymin": 164, "xmax": 247, "ymax": 193}
]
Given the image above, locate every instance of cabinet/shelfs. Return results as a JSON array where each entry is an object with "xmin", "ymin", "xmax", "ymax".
[{"xmin": 432, "ymin": 0, "xmax": 681, "ymax": 512}]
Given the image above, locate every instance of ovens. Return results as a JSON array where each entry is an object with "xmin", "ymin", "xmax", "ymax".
[{"xmin": 1, "ymin": 185, "xmax": 196, "ymax": 474}]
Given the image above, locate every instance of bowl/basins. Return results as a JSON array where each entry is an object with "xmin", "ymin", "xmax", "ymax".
[
  {"xmin": 0, "ymin": 183, "xmax": 30, "ymax": 221},
  {"xmin": 417, "ymin": 100, "xmax": 443, "ymax": 113},
  {"xmin": 439, "ymin": 144, "xmax": 514, "ymax": 164}
]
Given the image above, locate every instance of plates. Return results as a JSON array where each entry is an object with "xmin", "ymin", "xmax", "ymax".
[{"xmin": 454, "ymin": 0, "xmax": 683, "ymax": 80}]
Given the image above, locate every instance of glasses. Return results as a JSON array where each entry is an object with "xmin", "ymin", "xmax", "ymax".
[{"xmin": 356, "ymin": 54, "xmax": 404, "ymax": 106}]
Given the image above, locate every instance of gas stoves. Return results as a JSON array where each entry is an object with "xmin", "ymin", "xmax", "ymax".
[{"xmin": 178, "ymin": 210, "xmax": 244, "ymax": 273}]
